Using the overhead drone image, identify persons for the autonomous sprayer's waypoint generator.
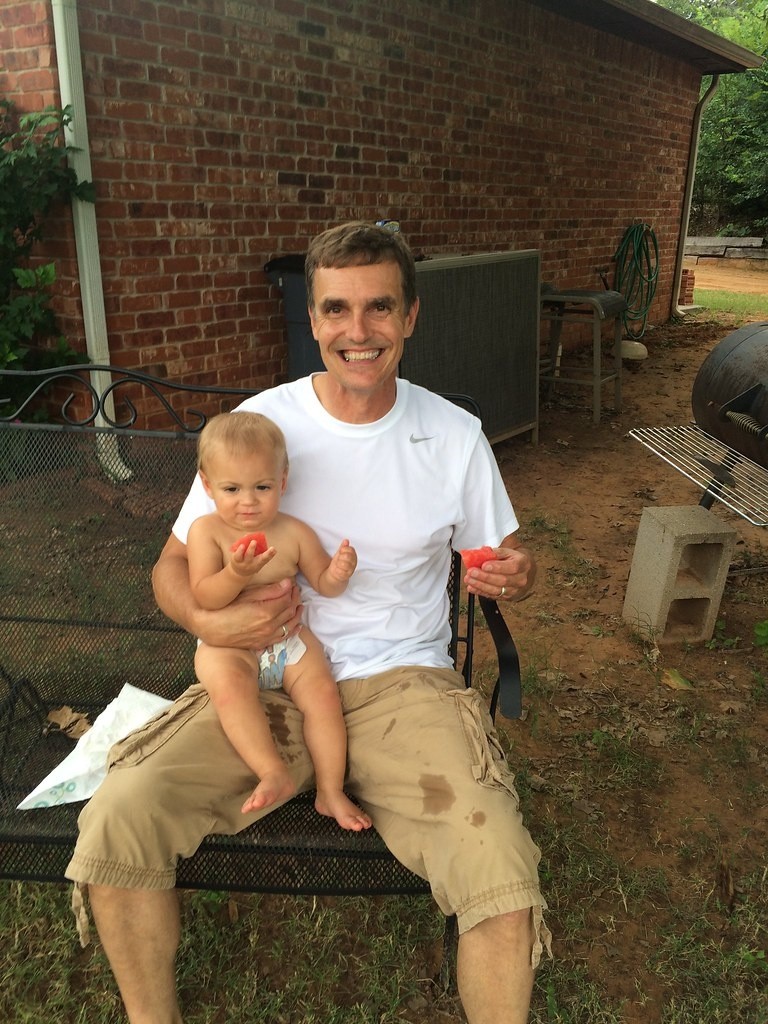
[63,222,556,1024]
[188,410,373,832]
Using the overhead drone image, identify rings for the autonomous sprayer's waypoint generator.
[498,587,506,596]
[281,625,290,638]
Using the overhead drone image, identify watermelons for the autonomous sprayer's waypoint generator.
[460,546,496,569]
[230,531,268,557]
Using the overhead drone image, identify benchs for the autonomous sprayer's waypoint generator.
[0,365,521,991]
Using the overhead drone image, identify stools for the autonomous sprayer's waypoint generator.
[541,287,627,421]
